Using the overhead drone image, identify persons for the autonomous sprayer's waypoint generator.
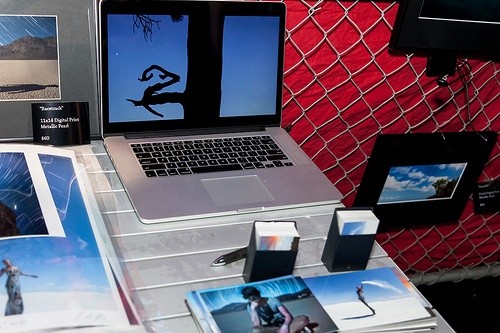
[356,284,376,314]
[0,258,38,317]
[241,286,310,333]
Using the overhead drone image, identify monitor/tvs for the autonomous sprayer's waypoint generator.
[387,0,500,62]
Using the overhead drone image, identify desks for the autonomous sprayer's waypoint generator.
[57,138,455,333]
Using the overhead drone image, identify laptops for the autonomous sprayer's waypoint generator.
[96,0,343,224]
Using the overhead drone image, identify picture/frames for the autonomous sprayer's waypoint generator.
[353,131,498,230]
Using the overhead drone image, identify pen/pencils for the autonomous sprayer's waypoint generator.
[210,244,249,268]
